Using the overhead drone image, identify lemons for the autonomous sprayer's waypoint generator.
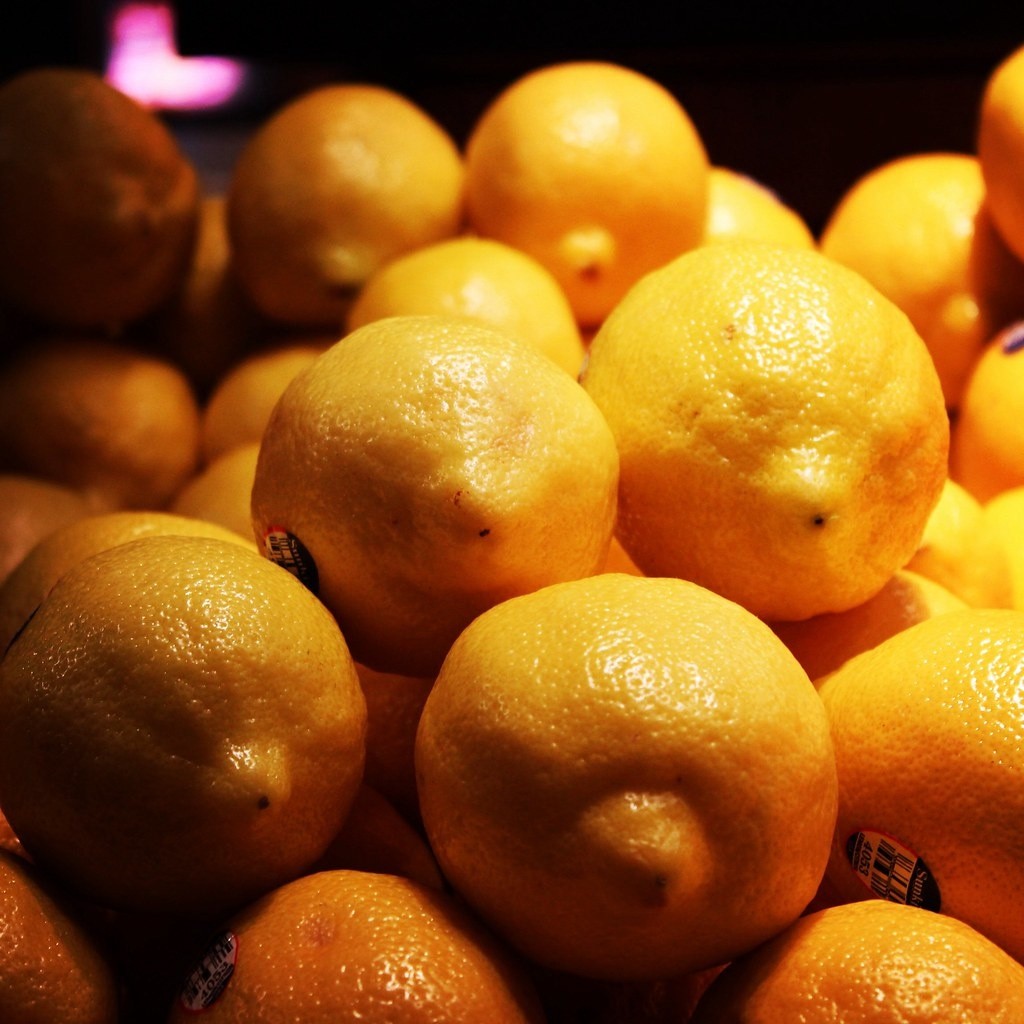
[0,44,1024,1024]
